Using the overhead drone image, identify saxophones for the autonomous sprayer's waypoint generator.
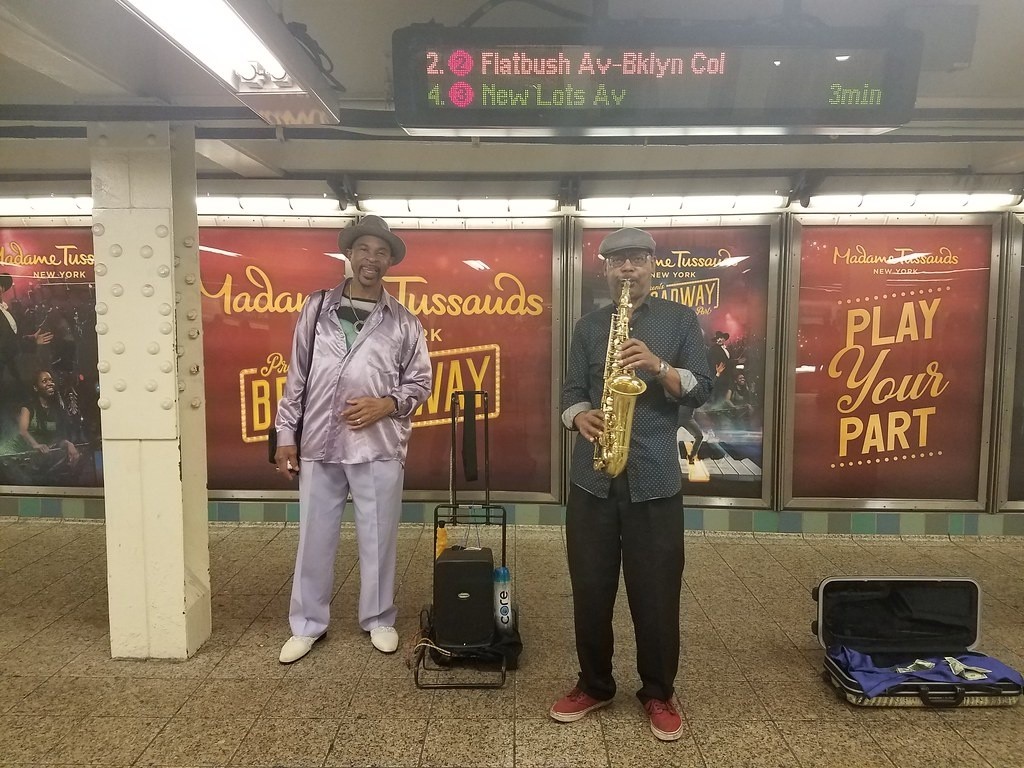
[593,280,648,477]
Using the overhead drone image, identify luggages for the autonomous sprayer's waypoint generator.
[811,575,1021,707]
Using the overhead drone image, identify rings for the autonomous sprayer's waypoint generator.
[357,419,362,425]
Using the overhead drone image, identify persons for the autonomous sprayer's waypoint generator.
[548,228,714,743]
[703,325,768,437]
[274,214,434,665]
[0,272,105,490]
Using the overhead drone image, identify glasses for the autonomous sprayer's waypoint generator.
[605,253,654,268]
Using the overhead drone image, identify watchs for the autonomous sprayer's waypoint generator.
[650,359,670,380]
[348,281,382,334]
[386,395,398,418]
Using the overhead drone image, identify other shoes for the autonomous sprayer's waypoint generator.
[370,625,399,652]
[279,631,327,663]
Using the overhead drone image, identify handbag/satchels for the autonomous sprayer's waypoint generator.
[268,407,304,466]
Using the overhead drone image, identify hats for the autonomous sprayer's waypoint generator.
[599,228,656,257]
[337,215,405,265]
[713,331,729,341]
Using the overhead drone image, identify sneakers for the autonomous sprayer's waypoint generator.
[550,685,613,721]
[642,696,683,740]
